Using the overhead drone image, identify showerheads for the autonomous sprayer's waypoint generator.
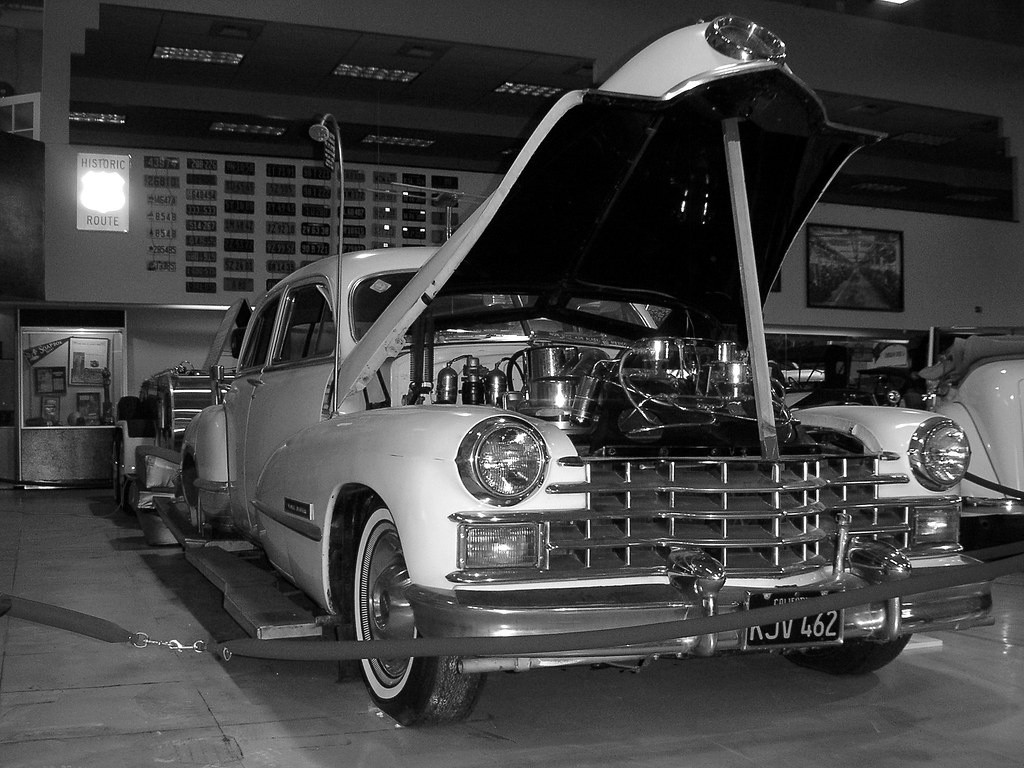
[308,110,351,420]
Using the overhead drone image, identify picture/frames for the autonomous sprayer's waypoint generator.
[68,337,110,386]
[805,221,906,313]
[41,395,61,423]
[34,366,67,396]
[78,392,101,417]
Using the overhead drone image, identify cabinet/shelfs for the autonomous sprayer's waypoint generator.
[15,306,129,488]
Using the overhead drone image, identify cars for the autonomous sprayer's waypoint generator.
[789,351,1024,528]
[178,26,994,731]
